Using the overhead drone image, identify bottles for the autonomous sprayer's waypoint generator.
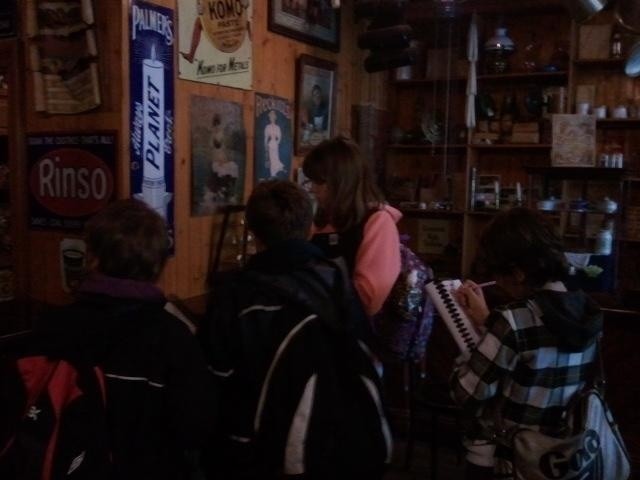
[611,33,624,56]
[595,228,613,255]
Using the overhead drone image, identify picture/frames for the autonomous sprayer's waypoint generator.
[268,0,341,53]
[294,54,338,157]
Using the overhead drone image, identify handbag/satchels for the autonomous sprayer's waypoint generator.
[497,386,635,480]
[348,207,436,364]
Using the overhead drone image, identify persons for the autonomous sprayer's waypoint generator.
[179,0,252,63]
[204,114,235,202]
[2,136,604,480]
[302,85,327,131]
[264,111,285,176]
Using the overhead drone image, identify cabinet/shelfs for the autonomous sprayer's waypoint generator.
[375,1,640,308]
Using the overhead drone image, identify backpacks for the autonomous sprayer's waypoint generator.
[254,257,395,480]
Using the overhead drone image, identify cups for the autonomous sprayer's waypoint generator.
[577,101,589,114]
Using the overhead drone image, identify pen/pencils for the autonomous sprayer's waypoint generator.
[453,281,497,296]
